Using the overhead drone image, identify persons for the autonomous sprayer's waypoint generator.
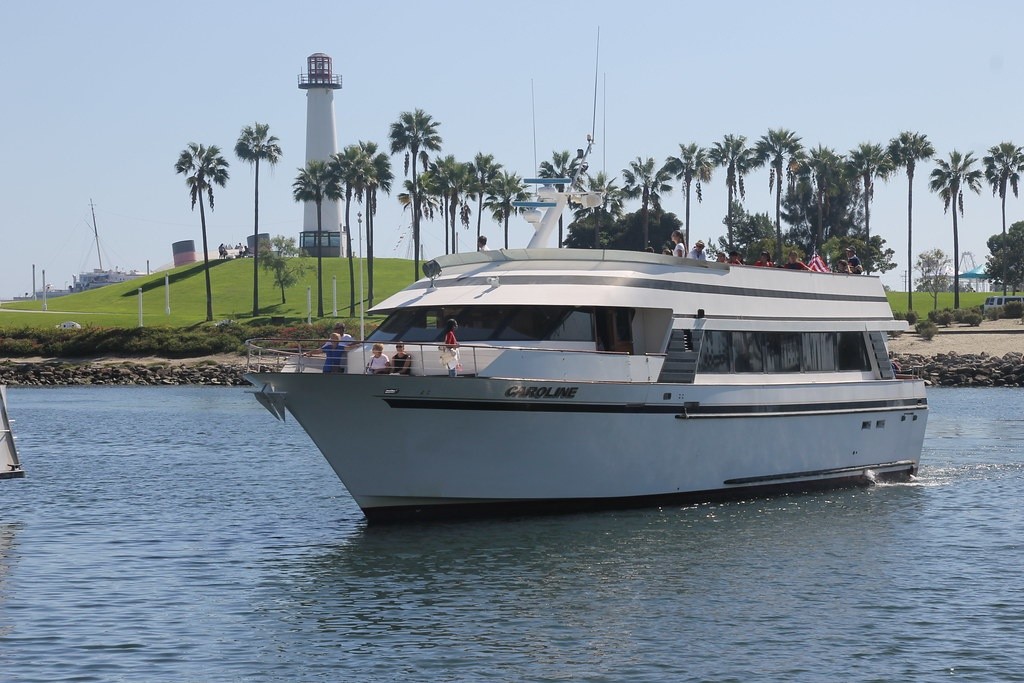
[368,343,390,375]
[444,319,460,377]
[321,323,354,374]
[477,229,863,277]
[391,341,413,375]
[306,332,363,374]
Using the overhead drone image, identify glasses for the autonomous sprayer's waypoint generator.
[696,245,701,247]
[396,345,402,348]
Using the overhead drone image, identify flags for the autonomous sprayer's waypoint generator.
[807,252,831,273]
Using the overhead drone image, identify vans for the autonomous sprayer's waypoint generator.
[983,296,1024,319]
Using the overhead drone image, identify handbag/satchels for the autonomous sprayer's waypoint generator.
[438,345,453,365]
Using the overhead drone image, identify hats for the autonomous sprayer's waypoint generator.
[695,240,705,248]
[845,245,856,254]
[761,251,770,262]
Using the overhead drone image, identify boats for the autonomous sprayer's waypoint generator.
[239,130,932,526]
